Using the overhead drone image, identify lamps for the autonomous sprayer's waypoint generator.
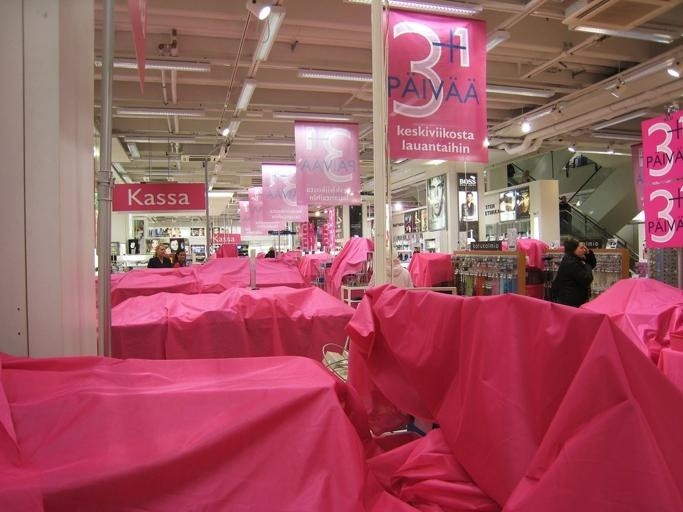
[204,1,290,193]
[362,53,681,192]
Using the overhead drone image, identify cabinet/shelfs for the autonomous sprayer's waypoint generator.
[450,251,526,296]
[540,248,631,301]
[481,180,560,245]
[391,206,428,264]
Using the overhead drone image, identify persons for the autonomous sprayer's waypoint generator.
[421,209,428,232]
[172,248,186,268]
[559,195,573,233]
[146,244,171,268]
[428,175,446,230]
[461,192,475,217]
[412,211,421,232]
[549,237,597,308]
[521,169,530,184]
